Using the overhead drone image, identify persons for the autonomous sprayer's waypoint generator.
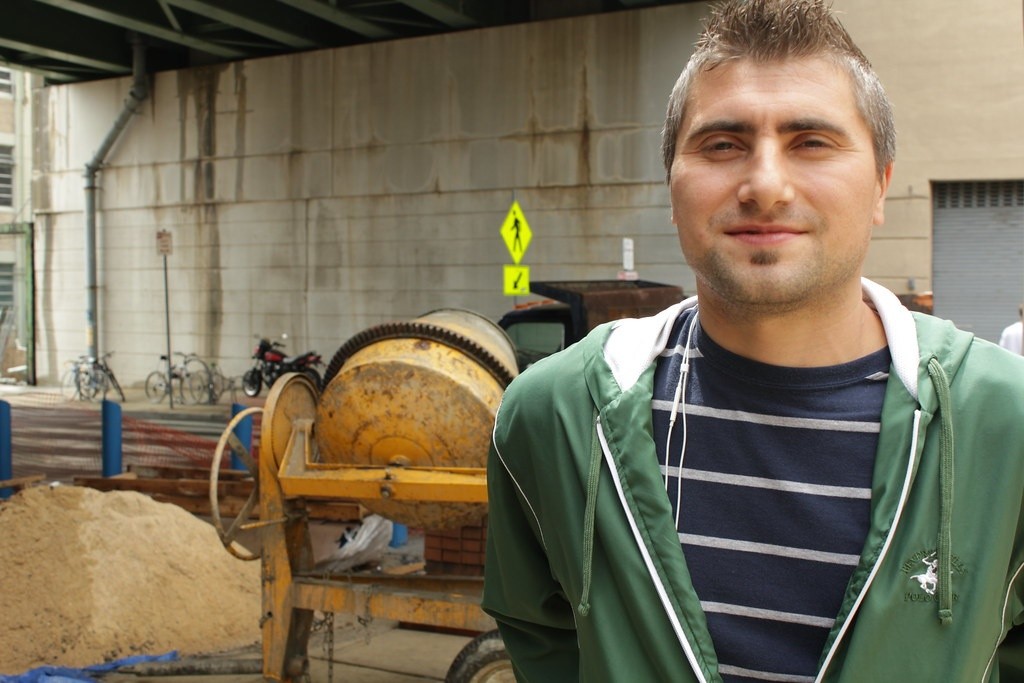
[999,305,1024,357]
[480,1,1024,683]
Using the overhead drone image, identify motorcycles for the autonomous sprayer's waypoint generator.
[241,332,324,389]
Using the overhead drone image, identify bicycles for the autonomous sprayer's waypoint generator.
[60,351,125,402]
[143,351,245,405]
